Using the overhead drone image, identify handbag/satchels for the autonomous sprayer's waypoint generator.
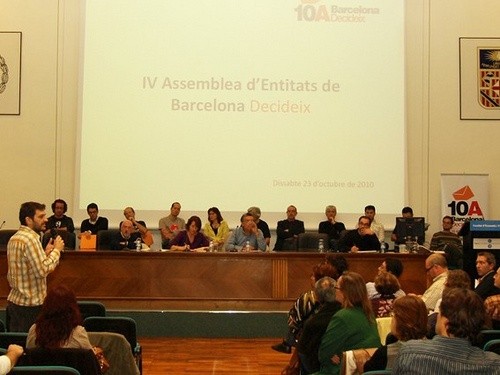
[93,345,110,372]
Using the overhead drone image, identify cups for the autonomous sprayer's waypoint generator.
[399,244,406,253]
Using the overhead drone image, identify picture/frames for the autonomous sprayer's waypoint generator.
[459,36,500,120]
[0,30,22,116]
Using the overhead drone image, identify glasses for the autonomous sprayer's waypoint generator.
[425,263,435,272]
[334,286,341,291]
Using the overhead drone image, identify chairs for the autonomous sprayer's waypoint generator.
[0,229,20,246]
[25,346,110,375]
[7,365,81,375]
[76,300,105,320]
[43,229,75,249]
[82,316,143,375]
[291,232,329,249]
[475,330,500,353]
[97,229,141,249]
[0,331,28,349]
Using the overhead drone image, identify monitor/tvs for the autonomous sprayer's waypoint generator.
[396,217,425,245]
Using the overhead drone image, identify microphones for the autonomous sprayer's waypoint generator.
[0,221,5,229]
[50,228,64,253]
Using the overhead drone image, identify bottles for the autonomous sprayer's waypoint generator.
[136,241,141,252]
[245,241,250,254]
[318,239,324,254]
[380,241,386,254]
[209,241,214,253]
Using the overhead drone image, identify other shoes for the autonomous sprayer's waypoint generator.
[271,343,291,354]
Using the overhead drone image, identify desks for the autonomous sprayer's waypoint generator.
[0,248,434,311]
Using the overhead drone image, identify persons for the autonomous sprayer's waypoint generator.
[365,205,385,244]
[26,287,110,375]
[224,212,267,253]
[203,207,230,248]
[240,206,271,247]
[271,244,500,354]
[158,202,187,249]
[430,216,463,251]
[363,295,432,373]
[389,287,500,375]
[44,199,153,249]
[311,270,381,375]
[391,206,429,242]
[318,204,345,240]
[272,205,305,251]
[0,344,24,375]
[167,215,209,251]
[336,216,381,252]
[281,276,343,375]
[6,202,65,333]
